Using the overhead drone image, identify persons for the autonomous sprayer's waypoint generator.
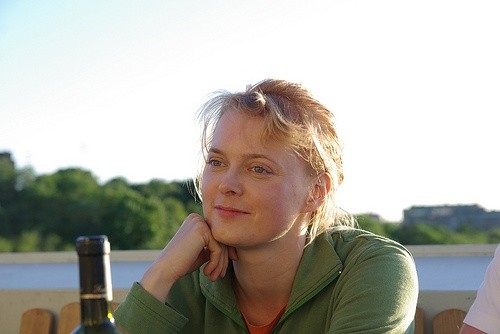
[459,242,500,334]
[112,79,420,334]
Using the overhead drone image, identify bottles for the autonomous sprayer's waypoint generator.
[71,235,121,334]
